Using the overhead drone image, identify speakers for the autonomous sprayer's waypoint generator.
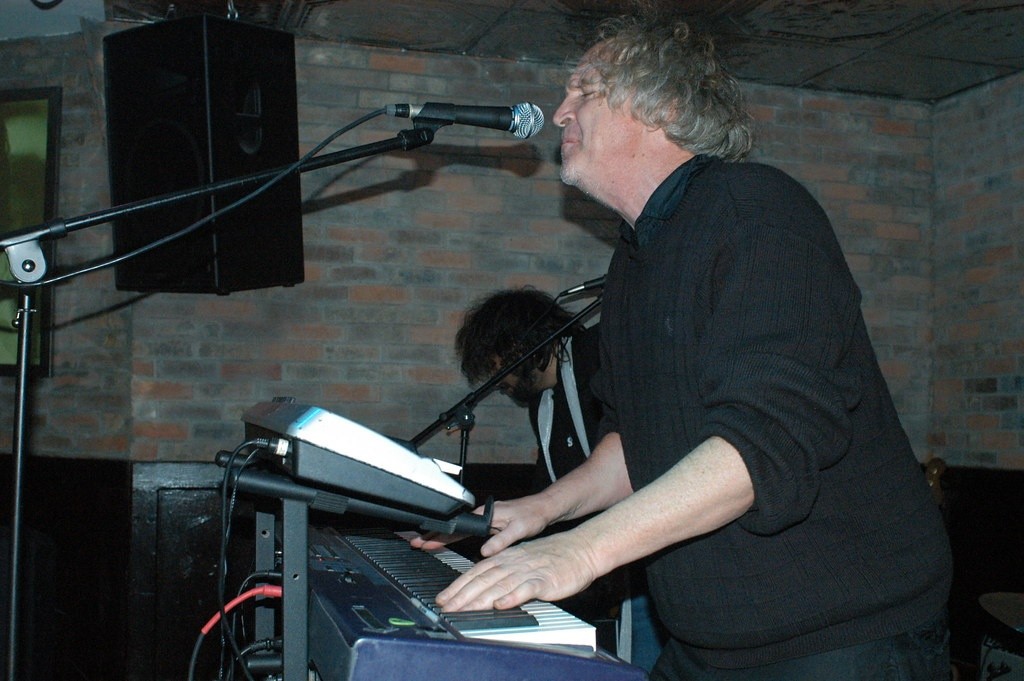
[103,14,305,297]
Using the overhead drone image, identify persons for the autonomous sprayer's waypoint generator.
[455,287,673,678]
[408,20,957,681]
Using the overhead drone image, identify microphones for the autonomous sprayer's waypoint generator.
[385,100,545,140]
[557,275,610,299]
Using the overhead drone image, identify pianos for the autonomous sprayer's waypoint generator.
[270,525,653,681]
